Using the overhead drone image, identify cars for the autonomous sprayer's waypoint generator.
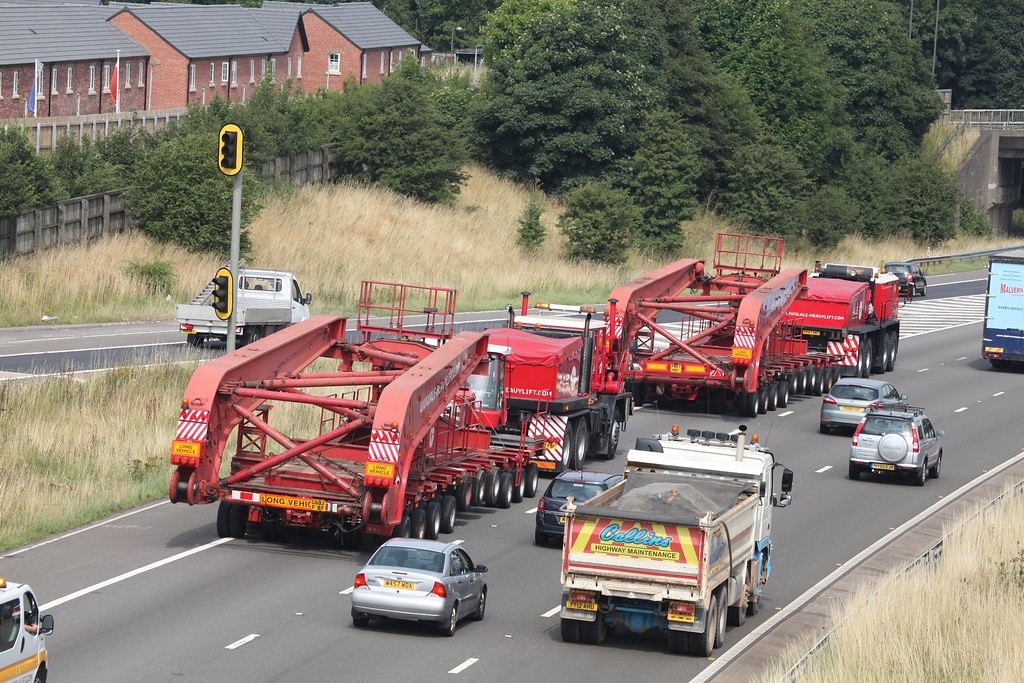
[820,378,908,434]
[884,262,927,296]
[351,536,489,636]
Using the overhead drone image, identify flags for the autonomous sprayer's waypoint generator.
[109,61,118,105]
[27,78,35,114]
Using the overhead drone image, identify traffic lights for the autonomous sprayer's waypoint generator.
[211,268,234,321]
[218,123,243,176]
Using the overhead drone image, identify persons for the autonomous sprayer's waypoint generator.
[24,623,38,633]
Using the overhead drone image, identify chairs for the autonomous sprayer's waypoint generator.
[381,557,398,566]
[426,563,441,573]
[254,285,262,290]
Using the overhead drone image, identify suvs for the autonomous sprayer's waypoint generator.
[849,403,944,488]
[535,470,624,545]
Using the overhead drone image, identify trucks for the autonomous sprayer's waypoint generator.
[981,249,1024,370]
[175,268,312,349]
[0,578,55,683]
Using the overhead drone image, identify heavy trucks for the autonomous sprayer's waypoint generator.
[560,424,794,658]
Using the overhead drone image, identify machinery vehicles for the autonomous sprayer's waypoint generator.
[611,231,901,417]
[168,279,632,549]
